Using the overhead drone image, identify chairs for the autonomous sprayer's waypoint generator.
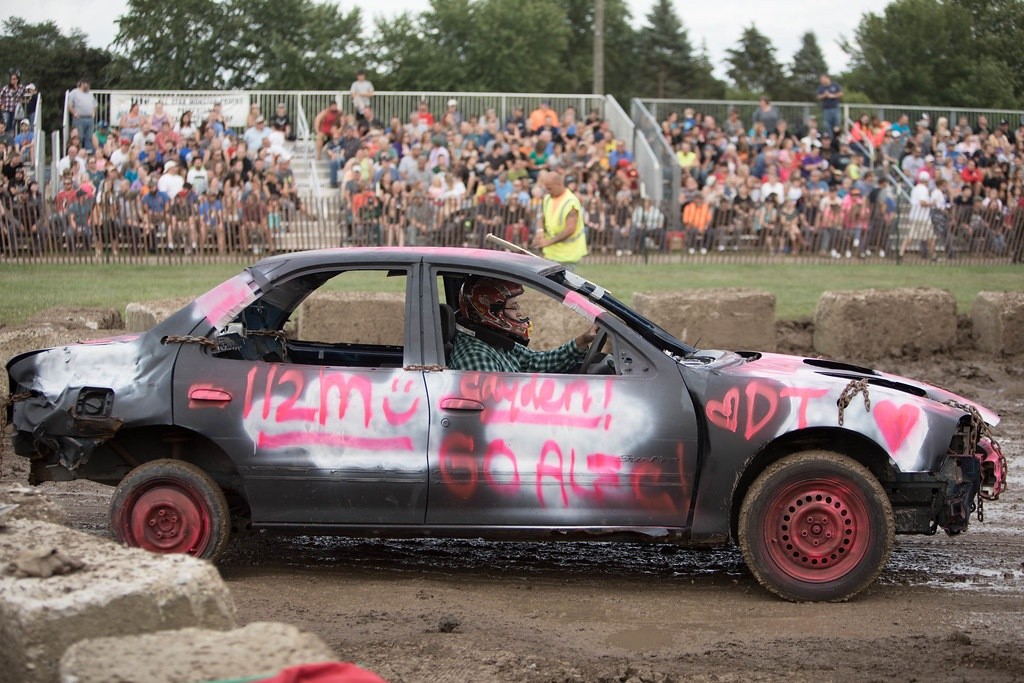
[440,303,456,361]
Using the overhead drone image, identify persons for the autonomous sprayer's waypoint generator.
[815,75,847,134]
[0,70,536,263]
[538,101,632,169]
[641,93,1023,266]
[530,170,590,276]
[566,170,668,255]
[449,271,604,375]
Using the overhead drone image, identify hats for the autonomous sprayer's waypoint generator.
[163,161,177,173]
[919,171,930,182]
[447,100,458,106]
[21,118,30,125]
[121,139,131,145]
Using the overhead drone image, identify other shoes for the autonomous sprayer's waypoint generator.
[865,249,872,256]
[853,238,859,247]
[616,249,622,256]
[837,253,841,258]
[718,244,724,251]
[701,247,707,255]
[845,249,851,258]
[626,250,632,256]
[879,250,885,258]
[688,247,695,254]
[831,249,837,258]
[859,252,865,258]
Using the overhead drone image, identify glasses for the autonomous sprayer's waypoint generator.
[145,141,153,145]
[64,183,70,185]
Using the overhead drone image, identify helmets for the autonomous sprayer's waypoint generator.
[459,275,532,347]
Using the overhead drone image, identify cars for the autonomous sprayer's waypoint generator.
[3,233,1008,603]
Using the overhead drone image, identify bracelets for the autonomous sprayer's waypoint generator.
[536,228,545,233]
